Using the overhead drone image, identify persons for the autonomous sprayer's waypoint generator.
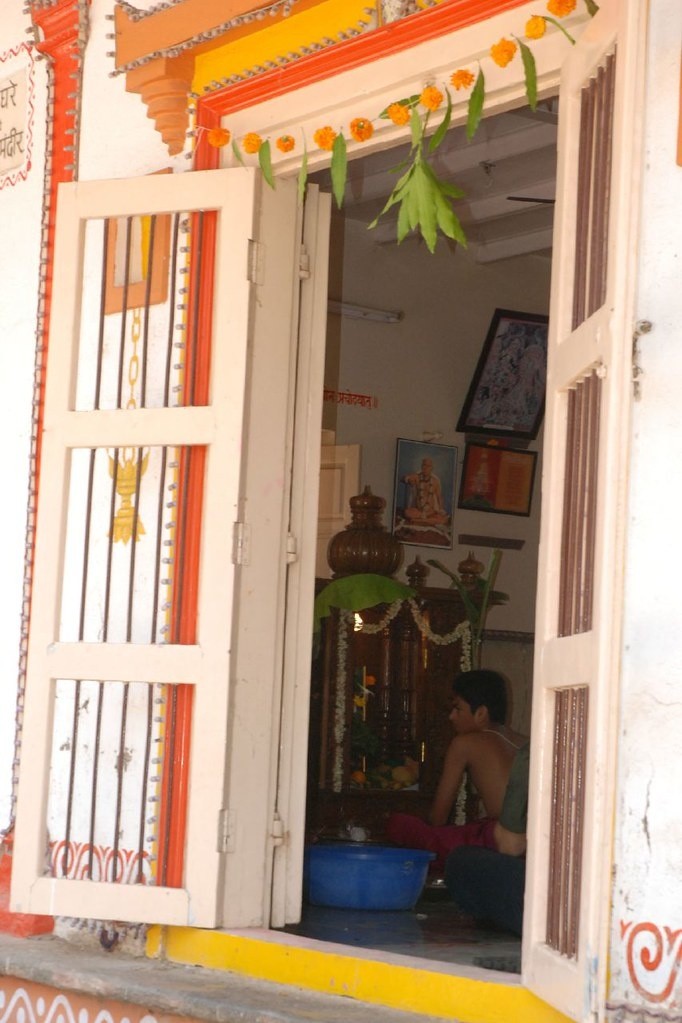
[384,668,530,883]
[447,735,530,937]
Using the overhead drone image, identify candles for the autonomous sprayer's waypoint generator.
[421,740,425,763]
[363,756,366,773]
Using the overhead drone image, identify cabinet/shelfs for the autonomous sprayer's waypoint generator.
[305,576,510,847]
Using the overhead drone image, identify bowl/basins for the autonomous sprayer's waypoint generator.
[304,844,436,911]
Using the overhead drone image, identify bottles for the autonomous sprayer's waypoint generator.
[327,484,403,579]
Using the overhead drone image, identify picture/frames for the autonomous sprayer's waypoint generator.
[392,437,459,550]
[455,309,548,441]
[456,442,538,517]
